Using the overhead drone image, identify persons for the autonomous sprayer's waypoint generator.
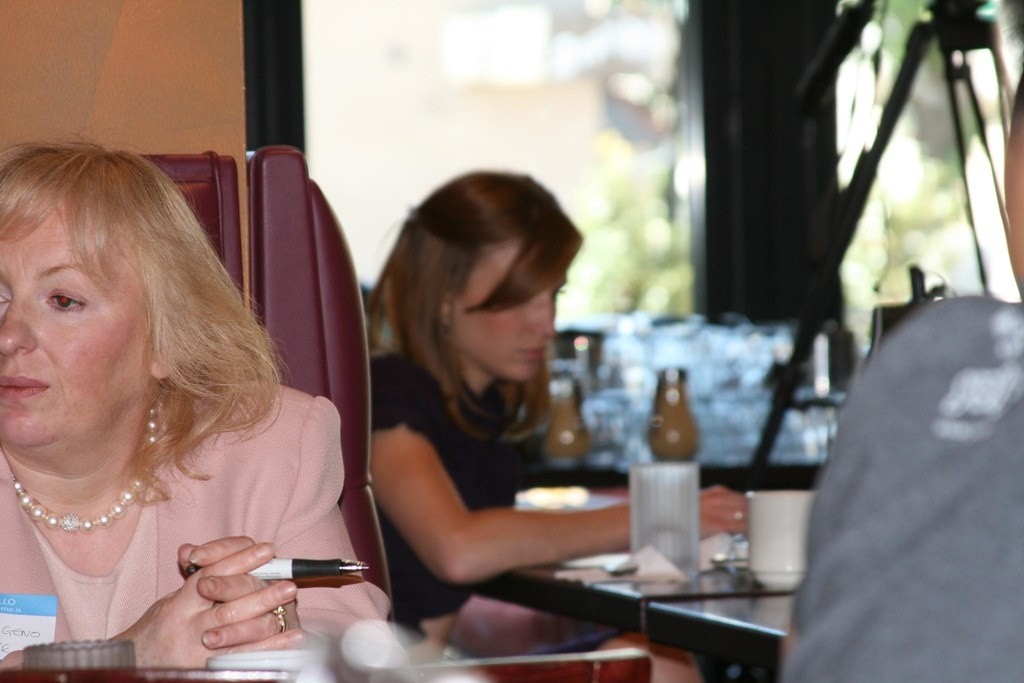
[1,146,393,673]
[773,49,1023,682]
[368,170,752,683]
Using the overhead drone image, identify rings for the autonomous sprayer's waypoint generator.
[733,509,742,521]
[268,605,288,633]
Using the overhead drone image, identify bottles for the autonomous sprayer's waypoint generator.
[541,372,588,459]
[644,365,699,461]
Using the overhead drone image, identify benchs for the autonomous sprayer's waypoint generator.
[245,141,655,683]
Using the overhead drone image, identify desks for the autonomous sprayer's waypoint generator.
[477,485,824,683]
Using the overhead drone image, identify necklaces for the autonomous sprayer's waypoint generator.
[10,407,159,534]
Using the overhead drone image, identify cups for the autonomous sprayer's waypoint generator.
[745,490,816,593]
[629,460,699,582]
[22,639,136,670]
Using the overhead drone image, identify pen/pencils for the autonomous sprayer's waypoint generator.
[186,558,370,579]
